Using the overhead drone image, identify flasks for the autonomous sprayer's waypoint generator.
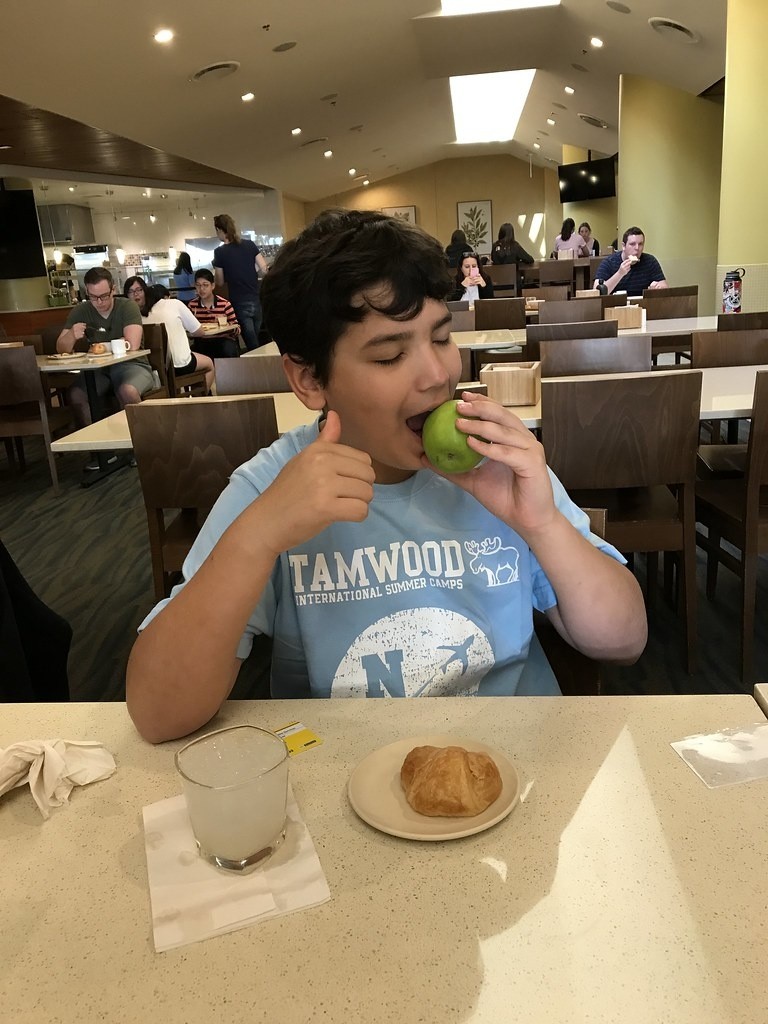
[722,268,746,314]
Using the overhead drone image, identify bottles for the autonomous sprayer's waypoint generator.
[595,279,608,296]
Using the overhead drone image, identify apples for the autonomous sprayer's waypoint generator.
[422,399,494,475]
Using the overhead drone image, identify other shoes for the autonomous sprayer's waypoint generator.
[85,454,117,470]
[131,461,137,466]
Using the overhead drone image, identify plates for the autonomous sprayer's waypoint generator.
[87,352,111,357]
[47,353,86,359]
[345,734,520,842]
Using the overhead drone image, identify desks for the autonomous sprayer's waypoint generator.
[0,695,768,1024]
[239,328,515,357]
[508,315,717,346]
[35,348,152,486]
[188,323,238,337]
[51,364,768,454]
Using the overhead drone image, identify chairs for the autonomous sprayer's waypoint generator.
[0,251,768,695]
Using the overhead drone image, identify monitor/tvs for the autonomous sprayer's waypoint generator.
[558,157,616,203]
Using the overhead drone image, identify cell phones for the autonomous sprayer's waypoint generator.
[470,268,479,279]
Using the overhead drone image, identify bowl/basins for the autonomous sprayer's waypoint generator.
[528,300,545,310]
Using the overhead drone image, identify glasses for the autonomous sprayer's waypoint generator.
[194,283,209,290]
[127,287,143,297]
[86,287,112,301]
[462,251,478,258]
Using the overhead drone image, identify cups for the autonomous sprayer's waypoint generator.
[111,339,130,355]
[175,724,291,875]
[218,317,227,326]
[526,297,536,305]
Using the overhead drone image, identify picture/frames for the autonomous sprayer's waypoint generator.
[457,200,494,255]
[380,206,416,224]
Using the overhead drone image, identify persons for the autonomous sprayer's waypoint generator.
[447,252,494,309]
[148,284,214,398]
[554,217,589,259]
[125,205,648,747]
[578,223,599,257]
[491,223,534,297]
[124,276,150,317]
[446,230,474,268]
[174,252,196,306]
[212,214,266,350]
[47,253,75,302]
[592,227,668,297]
[188,269,241,365]
[56,267,154,472]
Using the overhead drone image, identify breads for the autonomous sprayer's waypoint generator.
[628,255,639,261]
[92,344,106,354]
[400,745,503,818]
